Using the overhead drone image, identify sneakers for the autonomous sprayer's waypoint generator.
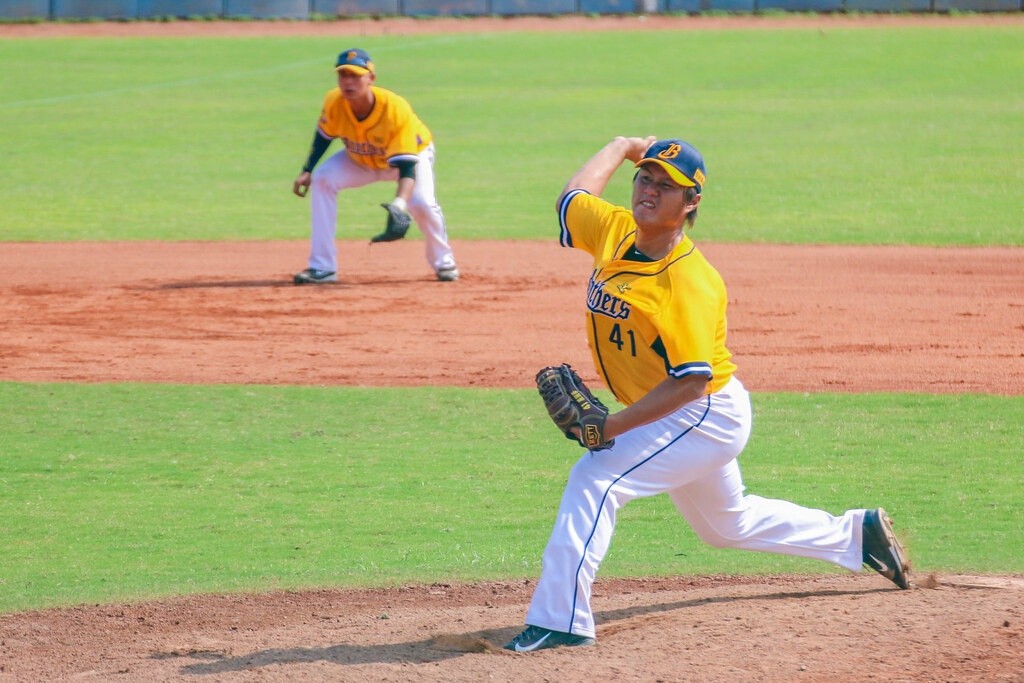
[862,507,911,589]
[503,626,596,652]
[294,268,337,283]
[437,266,458,281]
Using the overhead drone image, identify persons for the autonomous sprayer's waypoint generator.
[502,136,912,653]
[293,48,458,282]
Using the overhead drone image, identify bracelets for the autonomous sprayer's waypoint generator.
[392,198,406,210]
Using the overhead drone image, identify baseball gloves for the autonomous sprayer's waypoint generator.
[533,360,618,459]
[368,202,412,248]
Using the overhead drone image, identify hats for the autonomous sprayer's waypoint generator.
[334,49,374,75]
[635,138,706,194]
